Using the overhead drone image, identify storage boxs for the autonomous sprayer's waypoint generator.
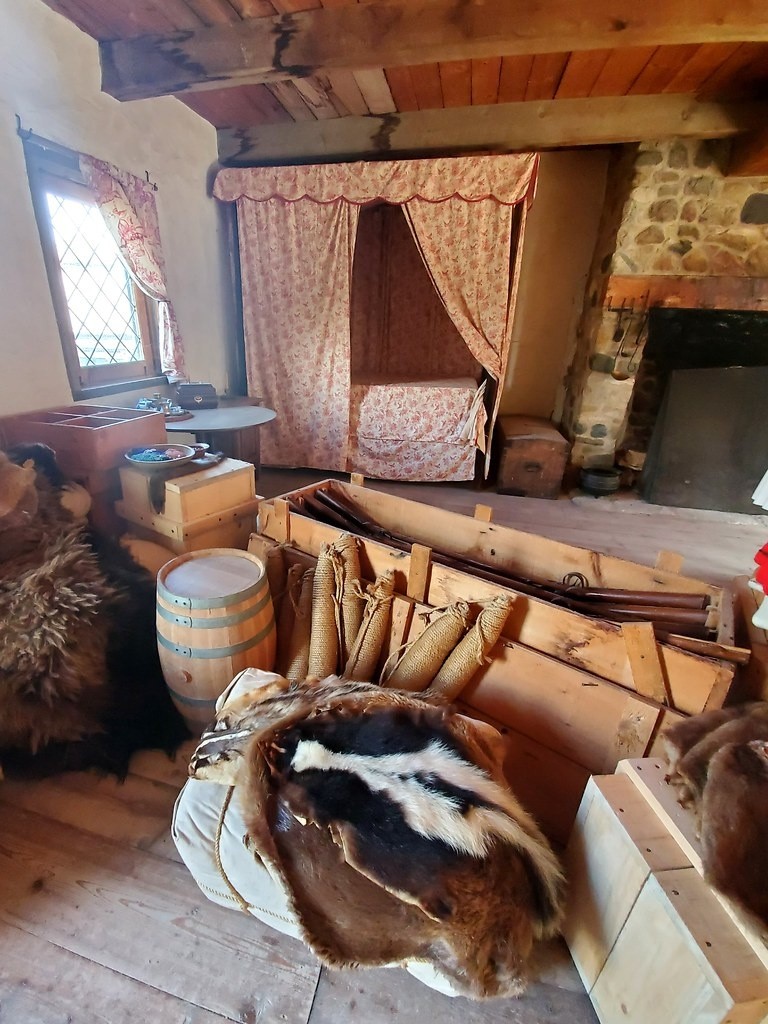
[244,476,735,835]
[0,404,264,581]
[561,757,768,1023]
[175,382,218,410]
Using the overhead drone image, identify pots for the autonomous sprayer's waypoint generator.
[619,459,642,489]
[580,465,622,499]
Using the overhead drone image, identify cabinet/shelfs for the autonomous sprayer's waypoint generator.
[206,395,264,479]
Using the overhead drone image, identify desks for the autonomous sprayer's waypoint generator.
[165,402,276,453]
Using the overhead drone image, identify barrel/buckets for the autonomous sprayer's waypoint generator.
[157,548,277,733]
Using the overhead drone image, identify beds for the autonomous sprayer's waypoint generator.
[212,149,541,482]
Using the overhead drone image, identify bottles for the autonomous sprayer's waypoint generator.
[137,393,184,417]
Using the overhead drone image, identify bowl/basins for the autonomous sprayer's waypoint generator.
[184,443,209,458]
[125,444,195,470]
